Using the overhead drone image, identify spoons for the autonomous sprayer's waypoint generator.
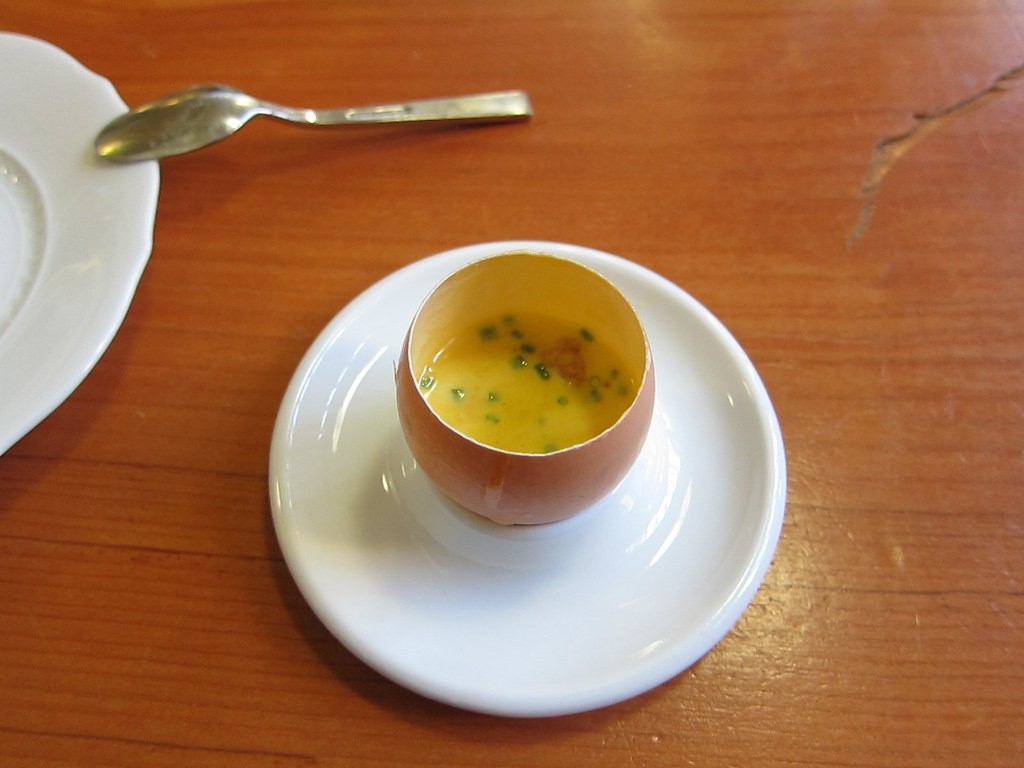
[93,82,534,162]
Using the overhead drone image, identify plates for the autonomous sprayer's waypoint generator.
[0,32,160,458]
[268,240,789,719]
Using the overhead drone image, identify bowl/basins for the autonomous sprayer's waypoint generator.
[396,249,655,527]
